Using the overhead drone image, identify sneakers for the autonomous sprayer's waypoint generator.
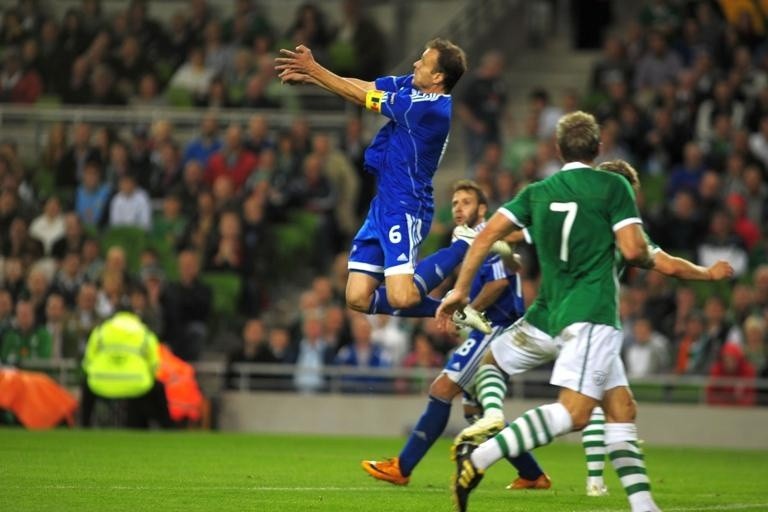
[450,413,505,512]
[441,288,493,335]
[451,223,512,257]
[506,473,552,491]
[586,485,608,496]
[361,457,411,486]
